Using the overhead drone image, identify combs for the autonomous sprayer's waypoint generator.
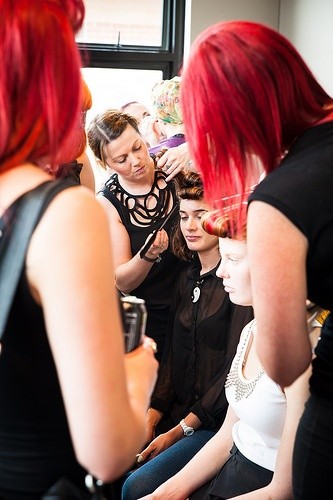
[140,204,178,259]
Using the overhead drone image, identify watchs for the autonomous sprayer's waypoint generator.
[180,418,194,437]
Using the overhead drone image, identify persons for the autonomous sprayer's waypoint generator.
[151,76,184,125]
[139,199,323,500]
[179,21,333,500]
[118,101,166,150]
[122,149,254,500]
[0,0,159,500]
[87,108,195,364]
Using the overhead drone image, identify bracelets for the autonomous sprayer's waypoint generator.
[139,250,158,263]
